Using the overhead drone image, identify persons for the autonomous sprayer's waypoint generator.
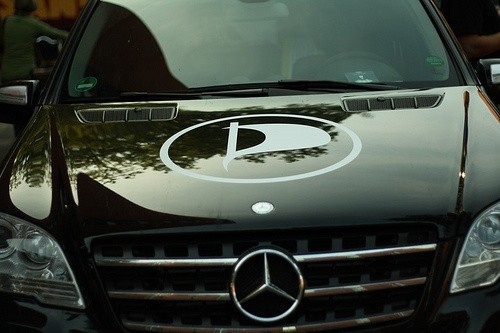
[0,0,72,137]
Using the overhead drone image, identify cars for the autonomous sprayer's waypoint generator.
[0,1,500,333]
